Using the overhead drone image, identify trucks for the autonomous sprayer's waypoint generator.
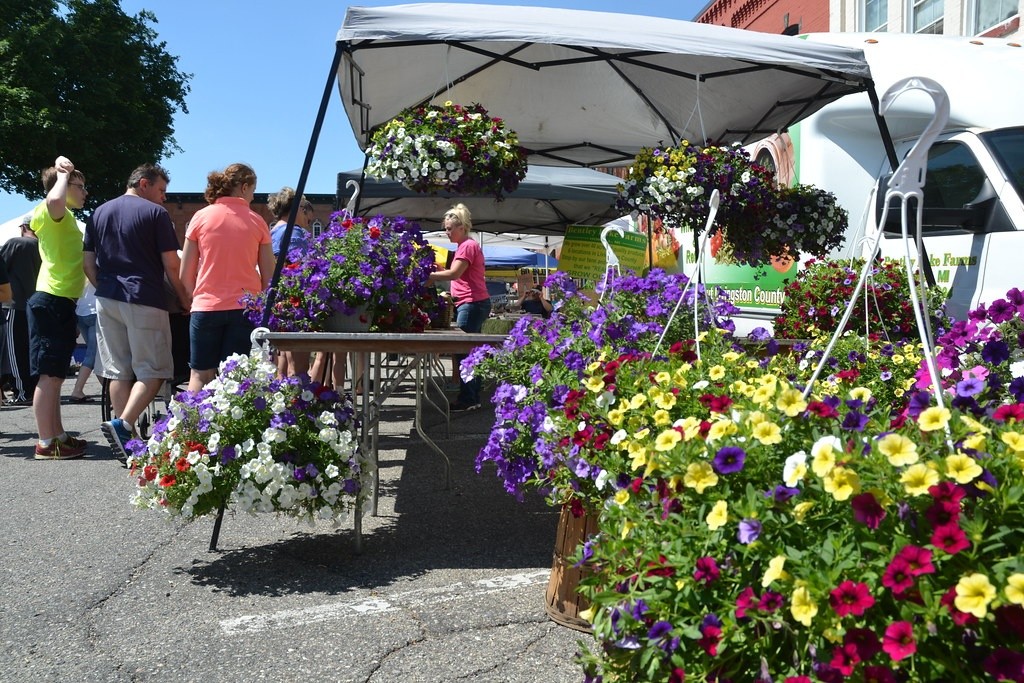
[659,30,1024,382]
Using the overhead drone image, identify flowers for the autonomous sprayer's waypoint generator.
[125,100,1024,683]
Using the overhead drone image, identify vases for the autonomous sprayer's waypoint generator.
[426,304,452,329]
[545,500,634,633]
[323,292,376,332]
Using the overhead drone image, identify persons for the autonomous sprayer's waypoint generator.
[427,203,492,412]
[178,163,275,394]
[261,186,313,379]
[0,215,41,409]
[84,164,191,467]
[70,273,99,406]
[311,351,364,396]
[514,284,554,320]
[26,156,88,461]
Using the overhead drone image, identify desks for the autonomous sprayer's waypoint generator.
[254,326,531,553]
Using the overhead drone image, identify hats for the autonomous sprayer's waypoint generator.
[18,215,33,227]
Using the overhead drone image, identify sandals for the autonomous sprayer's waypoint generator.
[69,395,95,403]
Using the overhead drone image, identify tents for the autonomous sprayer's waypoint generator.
[423,240,538,284]
[0,199,87,246]
[336,165,655,272]
[209,3,955,550]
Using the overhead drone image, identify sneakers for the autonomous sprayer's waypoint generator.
[450,400,481,413]
[100,418,133,464]
[35,432,88,460]
[9,399,33,406]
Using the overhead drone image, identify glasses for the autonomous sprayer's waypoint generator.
[68,183,85,190]
[300,205,307,212]
